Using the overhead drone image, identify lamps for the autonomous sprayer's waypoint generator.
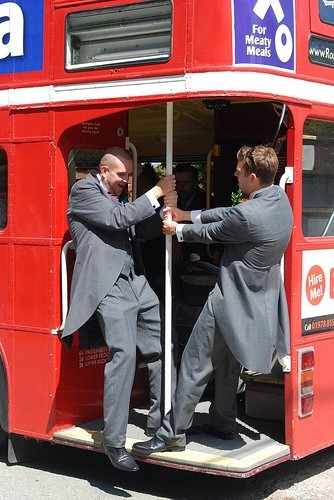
[202,99,230,111]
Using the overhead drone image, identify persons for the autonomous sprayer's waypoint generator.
[121,163,214,331]
[132,146,294,452]
[62,146,178,472]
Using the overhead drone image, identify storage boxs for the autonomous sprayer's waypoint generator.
[245,382,285,423]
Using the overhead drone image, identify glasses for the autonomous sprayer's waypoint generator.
[245,146,260,178]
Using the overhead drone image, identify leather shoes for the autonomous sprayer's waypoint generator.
[101,443,139,471]
[145,424,199,434]
[202,423,233,440]
[132,436,185,453]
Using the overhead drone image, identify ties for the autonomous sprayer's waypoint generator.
[108,192,123,207]
